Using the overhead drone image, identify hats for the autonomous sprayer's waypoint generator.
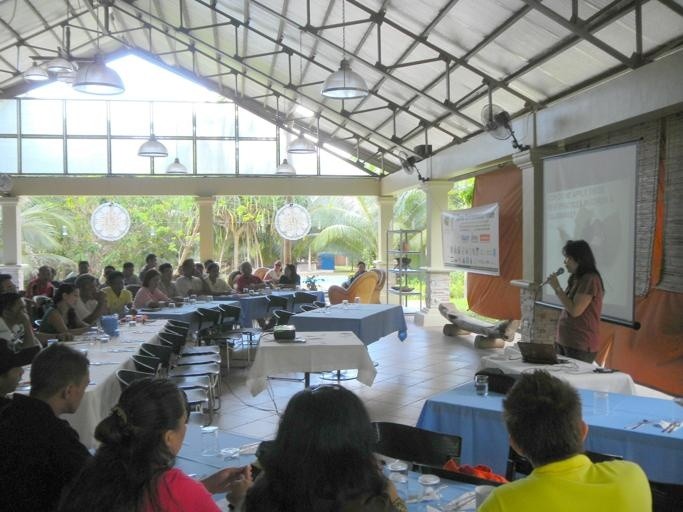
[0,338,40,374]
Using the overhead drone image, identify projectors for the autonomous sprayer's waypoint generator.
[273,324,296,341]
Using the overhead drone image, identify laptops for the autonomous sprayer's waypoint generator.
[517,341,569,365]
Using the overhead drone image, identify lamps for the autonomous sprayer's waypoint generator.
[275,87,295,174]
[167,135,189,175]
[41,23,77,74]
[138,114,168,157]
[287,27,315,154]
[72,1,126,97]
[322,1,372,100]
[24,48,47,82]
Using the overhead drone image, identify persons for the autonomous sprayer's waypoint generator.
[0,293,43,351]
[57,377,253,511]
[122,262,137,285]
[62,261,99,289]
[205,263,234,296]
[0,343,94,512]
[475,369,653,512]
[75,274,107,326]
[26,265,56,298]
[233,261,265,292]
[548,240,605,364]
[280,263,300,288]
[100,271,133,314]
[0,338,40,405]
[203,260,213,276]
[239,382,407,511]
[175,258,203,297]
[193,262,203,277]
[265,260,283,283]
[0,274,71,342]
[100,266,115,288]
[39,284,97,334]
[139,253,157,281]
[173,266,183,281]
[134,267,183,308]
[341,262,368,289]
[158,263,177,299]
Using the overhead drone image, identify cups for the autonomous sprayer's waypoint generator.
[207,295,213,305]
[594,391,609,414]
[191,295,196,300]
[200,426,221,457]
[387,459,409,503]
[126,314,133,326]
[343,299,348,311]
[46,338,58,345]
[184,297,189,305]
[266,287,271,294]
[159,301,164,311]
[169,303,176,314]
[136,315,145,331]
[87,332,97,347]
[129,320,136,334]
[102,338,110,354]
[244,288,249,294]
[250,290,254,297]
[419,475,441,512]
[475,374,490,397]
[190,299,197,309]
[355,297,361,310]
[115,329,122,343]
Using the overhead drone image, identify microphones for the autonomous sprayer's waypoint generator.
[537,266,565,290]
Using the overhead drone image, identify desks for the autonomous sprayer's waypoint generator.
[426,375,682,488]
[481,341,631,394]
[85,420,488,512]
[257,330,364,387]
[139,299,239,338]
[289,301,402,381]
[6,319,167,454]
[213,288,324,324]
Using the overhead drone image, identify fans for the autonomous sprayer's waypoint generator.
[398,150,430,181]
[481,103,530,152]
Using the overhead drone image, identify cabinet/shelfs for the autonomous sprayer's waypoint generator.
[387,230,422,315]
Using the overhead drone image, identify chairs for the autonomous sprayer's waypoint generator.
[158,332,221,375]
[297,304,317,313]
[194,307,220,346]
[313,300,325,308]
[162,324,221,373]
[218,303,240,323]
[114,370,213,426]
[271,309,295,325]
[328,273,378,304]
[369,268,385,292]
[265,295,287,312]
[131,355,215,413]
[167,320,221,354]
[291,291,316,313]
[139,343,220,390]
